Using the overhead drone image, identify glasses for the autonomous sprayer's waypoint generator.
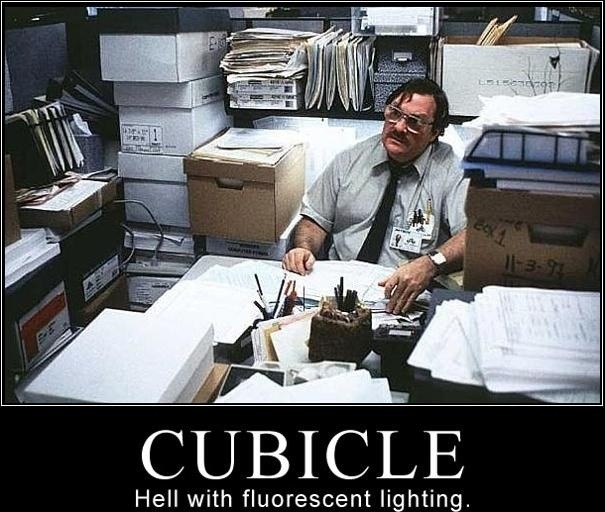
[383,102,435,138]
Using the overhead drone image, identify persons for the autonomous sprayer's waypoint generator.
[281,79,470,315]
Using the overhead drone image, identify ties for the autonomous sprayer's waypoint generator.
[352,162,416,265]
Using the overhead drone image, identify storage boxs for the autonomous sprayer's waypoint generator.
[461,179,599,297]
[2,4,306,403]
[441,38,598,118]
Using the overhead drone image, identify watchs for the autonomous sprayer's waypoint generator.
[427,249,449,276]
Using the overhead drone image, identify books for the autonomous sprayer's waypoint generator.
[220,27,378,115]
[476,15,518,46]
[250,305,323,367]
[286,256,434,315]
[1,65,120,208]
[455,91,601,201]
[404,284,603,406]
[191,126,306,168]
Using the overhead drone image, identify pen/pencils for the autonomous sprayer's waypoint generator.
[334,277,359,312]
[250,273,306,320]
